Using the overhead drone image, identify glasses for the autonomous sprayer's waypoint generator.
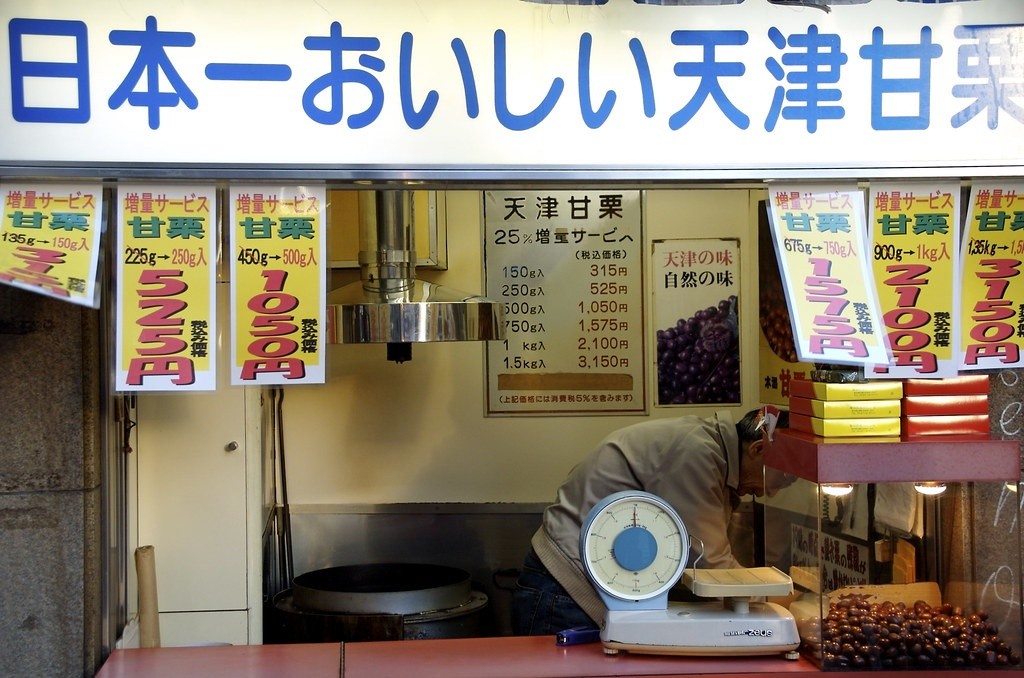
[778,470,793,489]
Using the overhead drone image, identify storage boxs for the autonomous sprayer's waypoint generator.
[787,374,989,439]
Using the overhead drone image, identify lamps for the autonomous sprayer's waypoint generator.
[821,482,853,497]
[914,482,947,495]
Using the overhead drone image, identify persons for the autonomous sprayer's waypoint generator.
[512,409,799,636]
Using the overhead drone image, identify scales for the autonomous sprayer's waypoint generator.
[578,489,803,661]
